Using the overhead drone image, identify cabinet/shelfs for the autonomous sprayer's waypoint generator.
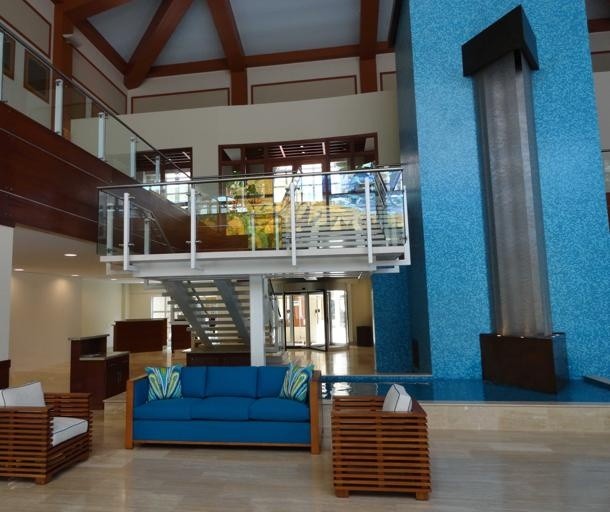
[69,333,130,410]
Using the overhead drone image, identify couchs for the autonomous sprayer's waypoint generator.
[124,366,323,455]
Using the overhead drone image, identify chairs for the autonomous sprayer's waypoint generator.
[1,380,95,486]
[242,166,303,250]
[329,384,432,501]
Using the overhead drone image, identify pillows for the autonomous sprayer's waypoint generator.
[144,362,184,402]
[279,362,315,401]
